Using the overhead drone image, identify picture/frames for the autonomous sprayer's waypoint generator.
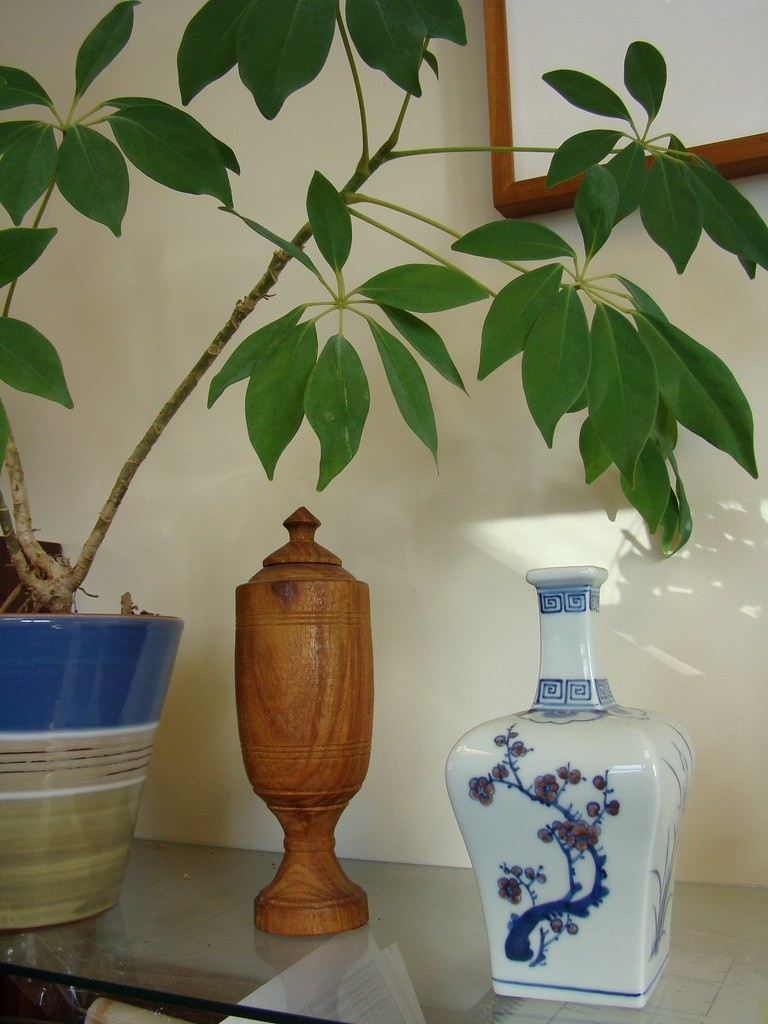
[482,0,768,217]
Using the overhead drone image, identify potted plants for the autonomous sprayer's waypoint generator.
[0,0,768,931]
